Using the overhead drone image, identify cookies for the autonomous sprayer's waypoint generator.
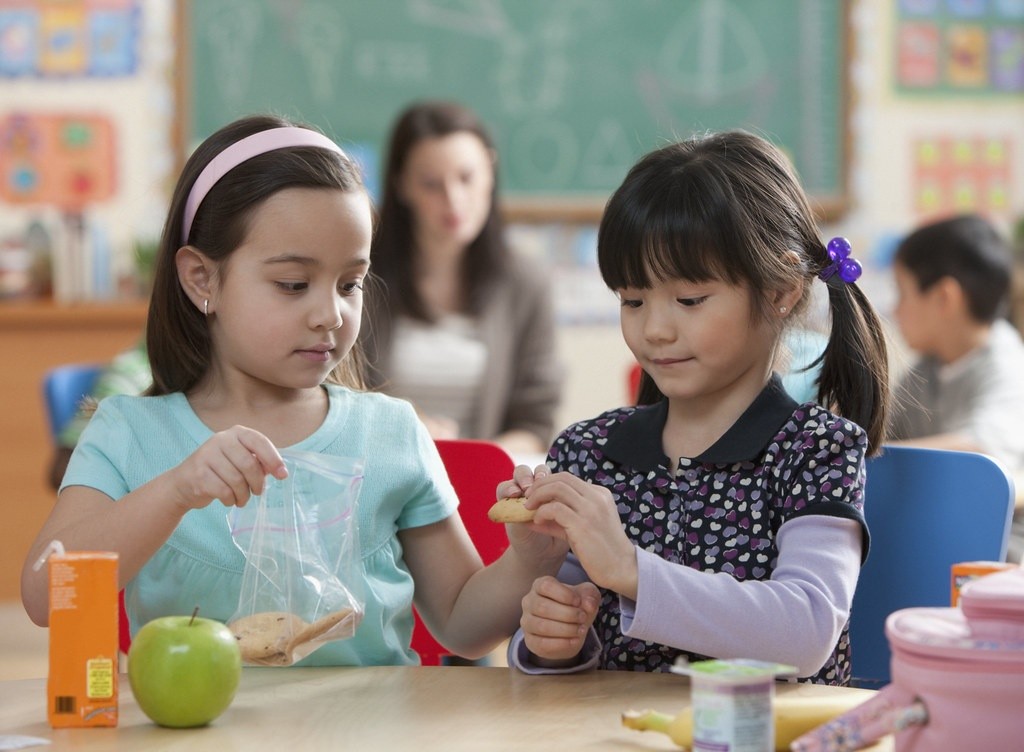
[225,612,301,657]
[286,609,351,653]
[243,621,308,666]
[487,497,540,523]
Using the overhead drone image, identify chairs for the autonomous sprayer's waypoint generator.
[852,448,1015,692]
[118,439,520,667]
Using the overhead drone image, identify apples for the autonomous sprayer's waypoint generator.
[129,607,240,727]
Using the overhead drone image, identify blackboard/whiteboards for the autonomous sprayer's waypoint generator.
[176,3,853,220]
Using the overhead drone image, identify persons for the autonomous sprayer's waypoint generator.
[355,96,567,458]
[18,113,571,670]
[504,126,892,687]
[882,208,1024,563]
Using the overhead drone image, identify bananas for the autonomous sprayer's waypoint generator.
[620,703,883,751]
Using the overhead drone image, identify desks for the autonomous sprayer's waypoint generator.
[0,668,695,752]
[0,292,189,600]
